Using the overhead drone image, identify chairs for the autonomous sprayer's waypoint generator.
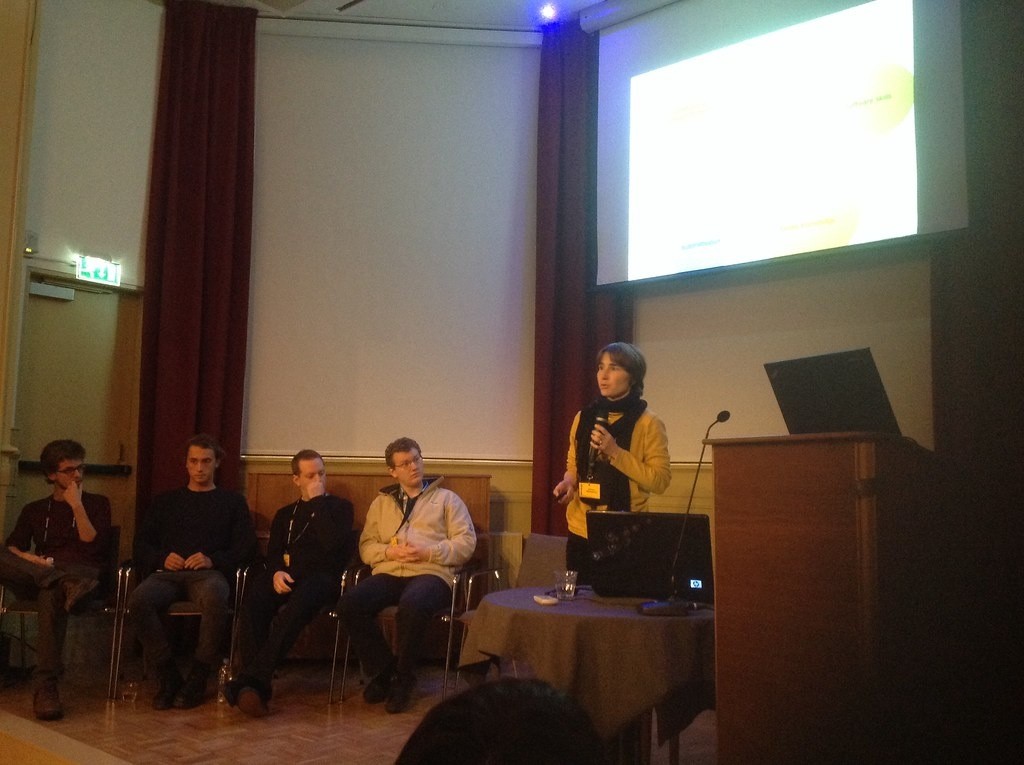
[339,523,476,705]
[229,528,362,705]
[113,509,258,697]
[0,524,130,699]
[455,532,570,697]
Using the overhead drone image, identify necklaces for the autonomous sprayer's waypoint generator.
[283,493,328,567]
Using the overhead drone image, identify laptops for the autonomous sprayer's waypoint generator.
[763,346,902,437]
[585,510,714,605]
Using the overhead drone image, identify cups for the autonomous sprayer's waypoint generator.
[122,681,139,703]
[552,571,577,600]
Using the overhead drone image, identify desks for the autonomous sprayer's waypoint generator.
[459,582,717,765]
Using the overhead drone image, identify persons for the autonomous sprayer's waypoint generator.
[0,438,113,721]
[552,341,672,586]
[342,437,478,713]
[218,450,355,717]
[124,435,257,710]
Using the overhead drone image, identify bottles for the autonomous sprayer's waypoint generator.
[218,659,233,703]
[46,557,53,565]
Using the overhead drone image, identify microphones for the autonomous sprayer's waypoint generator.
[590,408,609,463]
[638,410,732,616]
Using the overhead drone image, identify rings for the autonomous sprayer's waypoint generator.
[600,440,603,444]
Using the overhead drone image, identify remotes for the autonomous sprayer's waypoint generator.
[534,595,558,606]
[553,491,567,503]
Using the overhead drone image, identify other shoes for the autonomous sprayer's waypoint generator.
[224,680,268,718]
[152,671,207,709]
[383,670,417,715]
[32,683,66,720]
[363,671,391,704]
[64,576,100,614]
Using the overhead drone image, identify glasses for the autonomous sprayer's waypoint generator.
[394,454,423,468]
[57,463,88,474]
